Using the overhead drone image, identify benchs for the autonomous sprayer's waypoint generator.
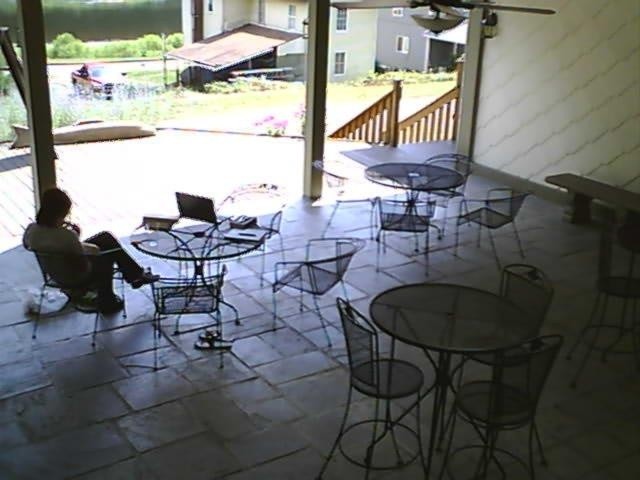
[544,174,640,225]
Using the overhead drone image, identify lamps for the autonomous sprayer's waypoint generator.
[481,11,499,39]
[410,7,464,35]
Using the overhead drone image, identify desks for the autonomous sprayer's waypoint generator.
[364,163,466,245]
[131,222,265,332]
[363,283,538,475]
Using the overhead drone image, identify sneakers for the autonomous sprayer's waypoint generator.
[132,272,160,288]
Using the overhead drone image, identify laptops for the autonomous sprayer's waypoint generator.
[176,192,234,225]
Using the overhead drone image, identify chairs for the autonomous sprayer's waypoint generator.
[30,249,103,350]
[311,295,425,480]
[419,150,473,235]
[566,218,640,391]
[497,264,554,338]
[275,239,364,347]
[147,267,226,367]
[444,332,564,478]
[459,186,534,267]
[312,158,382,240]
[209,184,288,288]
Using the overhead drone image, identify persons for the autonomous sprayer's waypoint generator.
[22,188,161,317]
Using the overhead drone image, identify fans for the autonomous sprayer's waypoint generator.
[327,1,555,34]
